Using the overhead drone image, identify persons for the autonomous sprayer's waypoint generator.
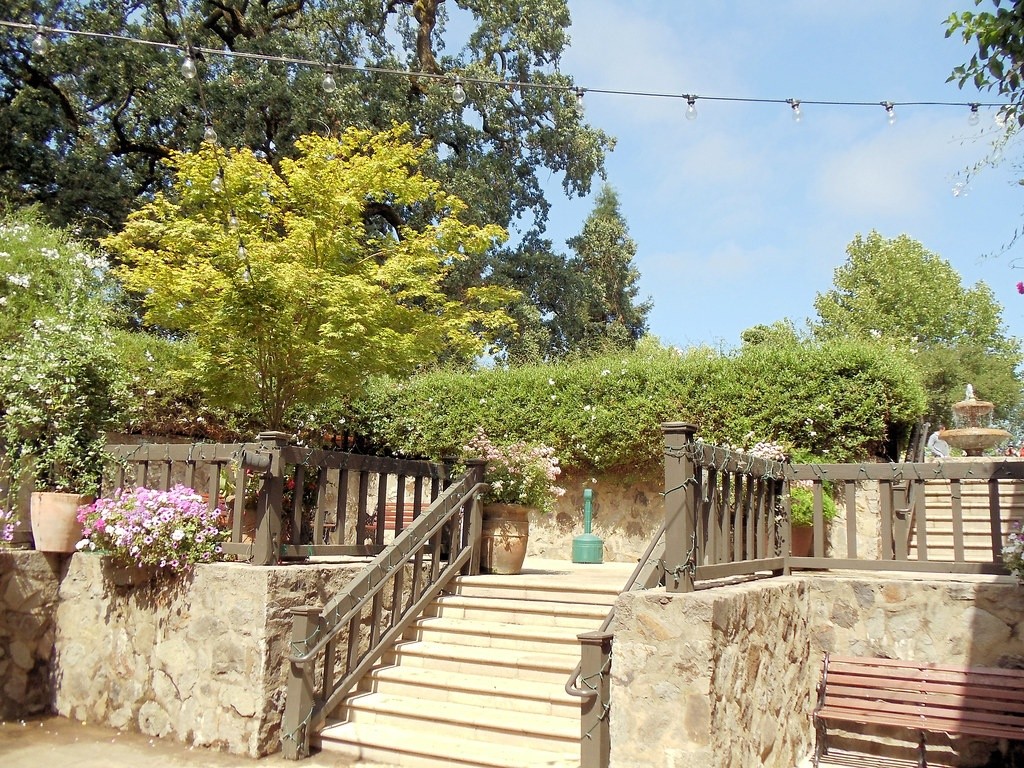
[927,424,950,457]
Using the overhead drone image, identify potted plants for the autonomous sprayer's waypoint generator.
[779,485,837,571]
[0,316,145,552]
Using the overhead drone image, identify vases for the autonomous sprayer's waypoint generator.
[482,502,529,574]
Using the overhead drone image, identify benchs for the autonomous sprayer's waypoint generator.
[807,650,1024,768]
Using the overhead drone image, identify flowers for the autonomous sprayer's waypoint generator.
[456,426,567,515]
[1000,520,1024,580]
[74,484,222,579]
